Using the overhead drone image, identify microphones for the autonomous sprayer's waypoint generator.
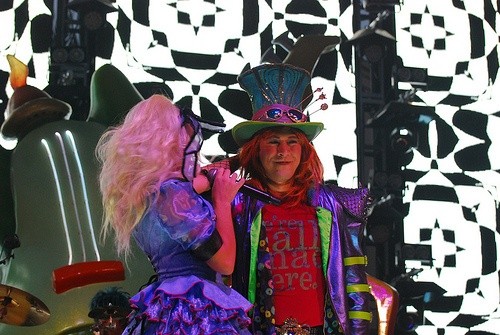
[206,168,282,207]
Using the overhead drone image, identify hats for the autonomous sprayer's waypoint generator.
[231,63,324,147]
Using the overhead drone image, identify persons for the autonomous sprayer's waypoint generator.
[94,94,256,335]
[200,62,374,334]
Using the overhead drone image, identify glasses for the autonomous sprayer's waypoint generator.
[255,107,308,123]
